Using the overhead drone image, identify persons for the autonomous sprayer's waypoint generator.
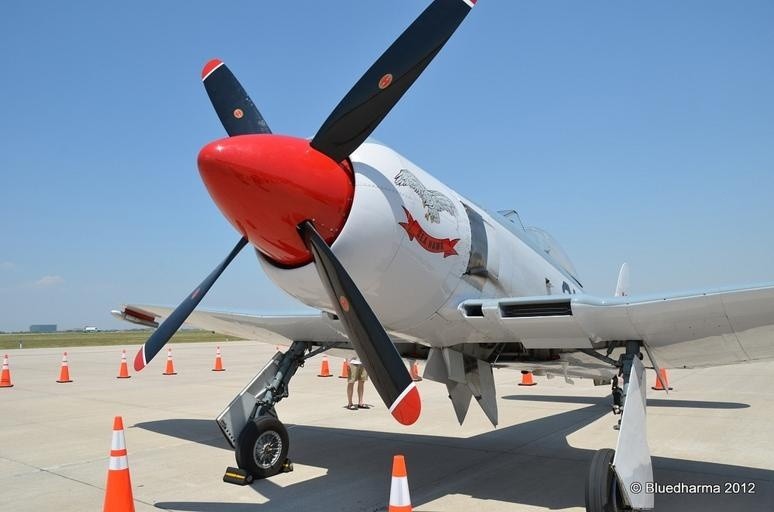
[345,348,370,410]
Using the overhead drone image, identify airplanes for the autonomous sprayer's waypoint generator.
[109,1,773,512]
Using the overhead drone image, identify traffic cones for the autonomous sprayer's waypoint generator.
[649,368,674,391]
[407,361,422,381]
[100,414,135,512]
[337,358,348,379]
[517,371,538,386]
[383,453,414,512]
[113,349,131,379]
[315,354,333,377]
[0,354,14,388]
[55,351,73,383]
[162,346,178,375]
[209,345,225,371]
